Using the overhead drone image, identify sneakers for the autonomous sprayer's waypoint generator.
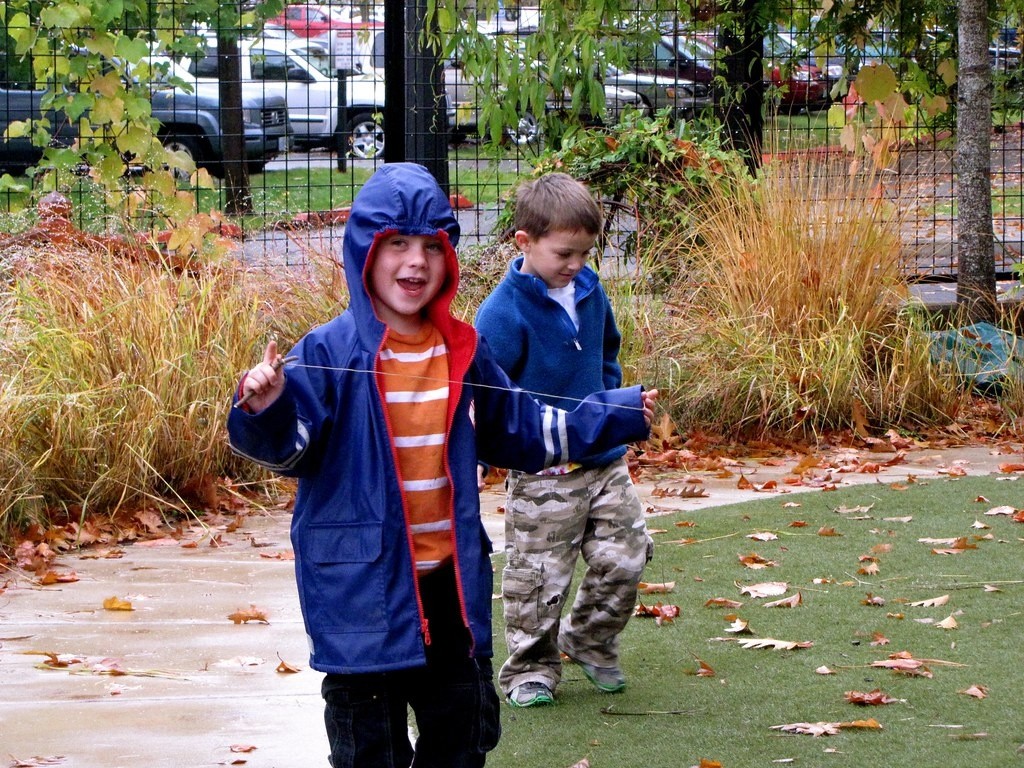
[559,643,627,692]
[509,682,555,708]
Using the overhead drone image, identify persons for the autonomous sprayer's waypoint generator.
[469,169,654,709]
[227,161,661,767]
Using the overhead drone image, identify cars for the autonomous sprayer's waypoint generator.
[0,37,296,183]
[158,0,1024,160]
